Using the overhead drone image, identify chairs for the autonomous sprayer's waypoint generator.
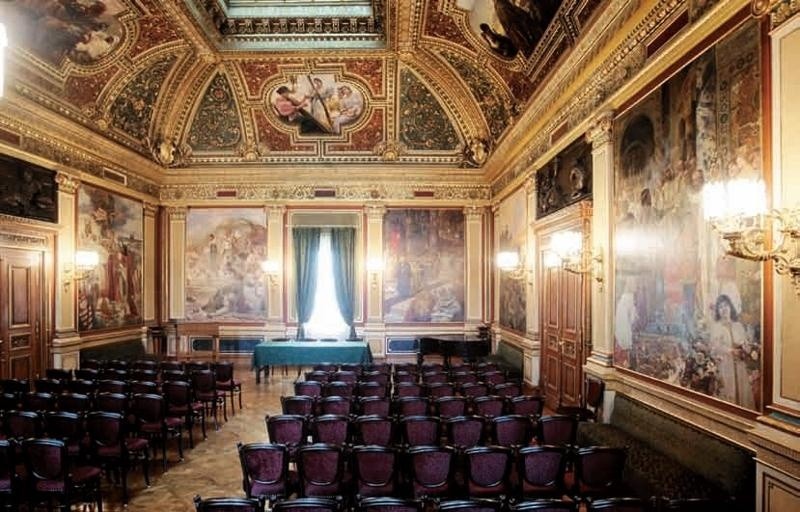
[0,360,242,512]
[193,361,709,511]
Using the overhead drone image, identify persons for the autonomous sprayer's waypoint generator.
[613,167,757,412]
[273,78,361,136]
[395,251,411,298]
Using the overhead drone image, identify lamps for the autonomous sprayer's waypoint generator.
[62,249,101,287]
[550,229,608,289]
[699,178,800,288]
[493,249,535,288]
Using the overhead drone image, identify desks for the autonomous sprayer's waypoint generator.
[174,320,222,360]
[250,341,375,385]
